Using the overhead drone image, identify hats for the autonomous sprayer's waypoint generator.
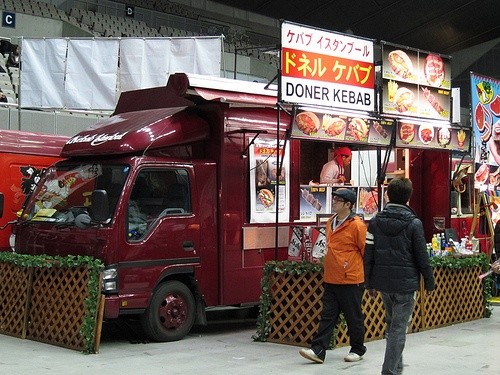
[333,189,356,204]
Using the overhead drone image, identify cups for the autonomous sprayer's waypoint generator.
[388,82,398,102]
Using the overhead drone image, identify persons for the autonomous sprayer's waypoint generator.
[299,190,368,363]
[320,146,352,183]
[363,177,434,375]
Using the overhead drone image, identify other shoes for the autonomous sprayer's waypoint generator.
[344,352,363,361]
[299,348,324,363]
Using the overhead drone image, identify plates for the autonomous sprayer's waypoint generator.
[437,128,452,148]
[419,123,434,145]
[399,124,416,145]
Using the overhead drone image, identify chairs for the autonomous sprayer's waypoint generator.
[159,182,189,211]
[0,0,280,117]
[107,182,122,212]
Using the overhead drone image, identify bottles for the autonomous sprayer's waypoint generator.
[425,232,480,257]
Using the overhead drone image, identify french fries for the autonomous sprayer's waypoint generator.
[457,130,466,142]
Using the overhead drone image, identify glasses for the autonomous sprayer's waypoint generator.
[334,198,345,203]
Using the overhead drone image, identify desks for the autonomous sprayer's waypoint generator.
[254,250,488,349]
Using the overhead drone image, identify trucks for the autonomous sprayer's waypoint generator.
[1,71,496,340]
